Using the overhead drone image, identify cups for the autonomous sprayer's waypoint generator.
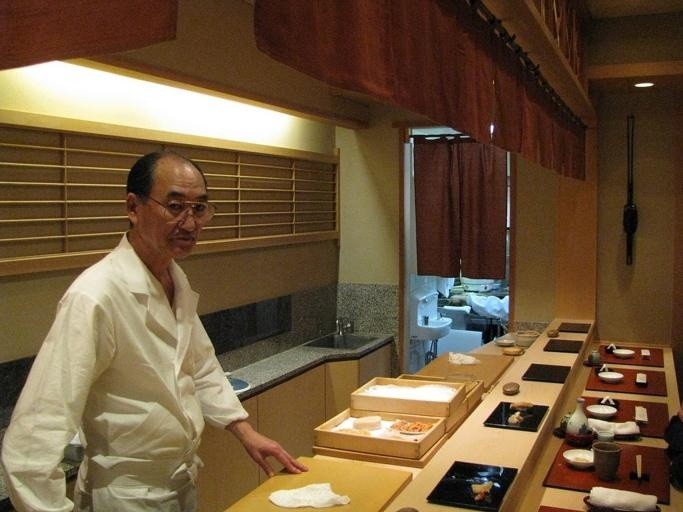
[592,441,623,480]
[597,431,613,442]
[565,429,593,446]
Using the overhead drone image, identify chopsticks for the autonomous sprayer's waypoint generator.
[606,343,616,349]
[599,364,608,371]
[635,454,641,478]
[601,395,615,405]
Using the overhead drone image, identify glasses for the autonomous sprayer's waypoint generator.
[136,190,215,226]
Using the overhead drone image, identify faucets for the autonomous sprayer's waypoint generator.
[336,318,352,336]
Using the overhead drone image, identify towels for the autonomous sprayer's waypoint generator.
[448,351,481,366]
[265,481,351,509]
[588,483,659,511]
[584,416,640,439]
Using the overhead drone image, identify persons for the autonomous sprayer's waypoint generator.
[0,150,309,512]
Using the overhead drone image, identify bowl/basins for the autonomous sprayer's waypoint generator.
[598,371,624,383]
[514,330,539,347]
[562,447,592,469]
[604,345,620,353]
[598,398,618,407]
[586,404,617,419]
[595,367,613,376]
[612,349,635,358]
[501,348,523,356]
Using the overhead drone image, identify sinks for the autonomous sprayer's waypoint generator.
[303,334,379,351]
[416,317,453,340]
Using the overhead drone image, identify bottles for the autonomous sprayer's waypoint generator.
[587,351,601,363]
[560,396,588,432]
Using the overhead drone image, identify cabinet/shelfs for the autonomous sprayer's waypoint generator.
[191,337,394,511]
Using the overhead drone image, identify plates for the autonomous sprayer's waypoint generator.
[493,339,515,347]
[635,406,648,422]
[635,373,646,383]
[582,494,661,511]
[553,427,565,437]
[582,360,603,366]
[641,349,650,359]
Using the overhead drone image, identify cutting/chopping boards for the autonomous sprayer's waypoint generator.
[311,409,469,469]
[413,351,514,392]
[224,455,413,512]
